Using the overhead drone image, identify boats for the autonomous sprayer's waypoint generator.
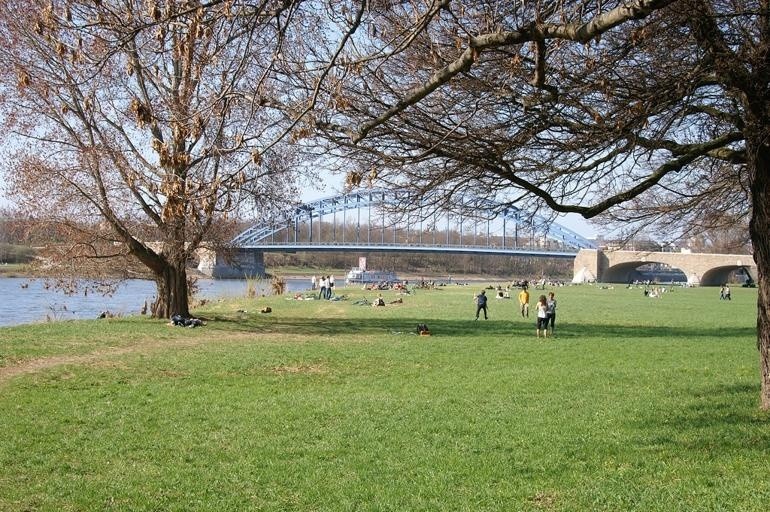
[344,266,403,287]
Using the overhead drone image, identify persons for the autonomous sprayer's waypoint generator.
[518,286,529,319]
[534,295,549,339]
[719,285,725,301]
[312,274,335,301]
[544,291,557,336]
[284,291,318,301]
[330,275,446,307]
[473,289,488,321]
[495,275,616,299]
[625,277,695,300]
[723,285,731,300]
